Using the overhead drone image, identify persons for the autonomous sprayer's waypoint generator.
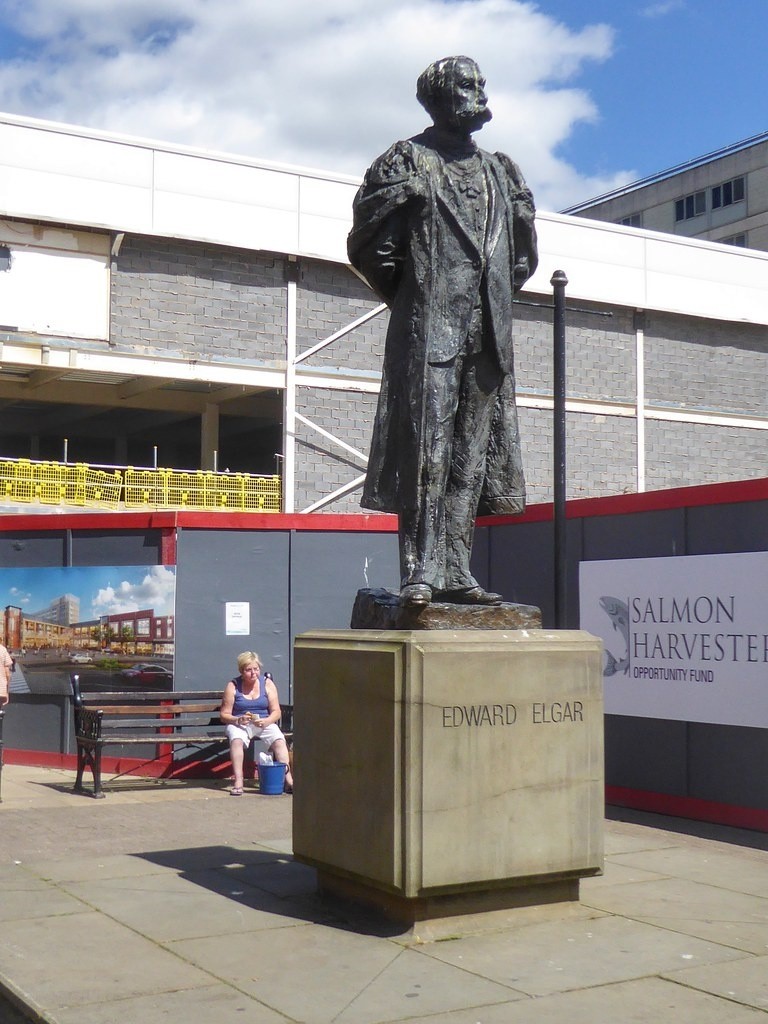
[220,651,293,795]
[21,644,135,660]
[0,644,13,706]
[344,54,540,606]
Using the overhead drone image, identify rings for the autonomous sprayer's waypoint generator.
[259,723,262,727]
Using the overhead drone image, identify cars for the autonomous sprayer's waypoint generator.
[120,664,172,686]
[68,654,93,664]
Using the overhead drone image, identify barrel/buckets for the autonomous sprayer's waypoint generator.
[257,762,288,795]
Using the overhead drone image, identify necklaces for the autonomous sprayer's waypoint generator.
[445,148,485,198]
[244,678,256,696]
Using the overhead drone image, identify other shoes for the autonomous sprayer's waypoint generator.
[230,787,244,795]
[286,784,293,794]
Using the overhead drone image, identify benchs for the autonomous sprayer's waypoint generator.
[73,674,294,799]
